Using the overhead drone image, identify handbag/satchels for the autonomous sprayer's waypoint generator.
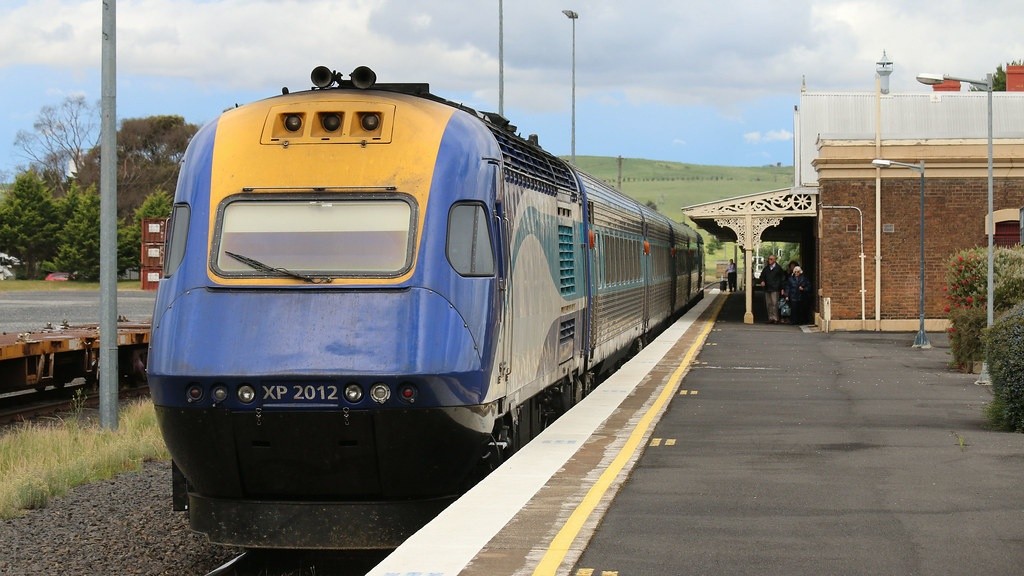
[781,300,791,315]
[778,294,785,309]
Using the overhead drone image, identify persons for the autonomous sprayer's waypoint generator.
[760,255,813,324]
[724,259,737,292]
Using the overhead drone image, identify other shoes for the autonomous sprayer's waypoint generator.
[769,316,779,323]
[781,319,791,323]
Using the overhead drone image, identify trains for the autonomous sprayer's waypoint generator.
[143,65,706,549]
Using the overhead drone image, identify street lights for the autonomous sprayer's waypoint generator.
[872,158,932,351]
[915,71,994,387]
[562,9,579,166]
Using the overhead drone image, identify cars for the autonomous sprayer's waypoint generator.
[44,271,78,281]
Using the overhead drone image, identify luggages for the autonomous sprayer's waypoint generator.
[720,274,727,292]
